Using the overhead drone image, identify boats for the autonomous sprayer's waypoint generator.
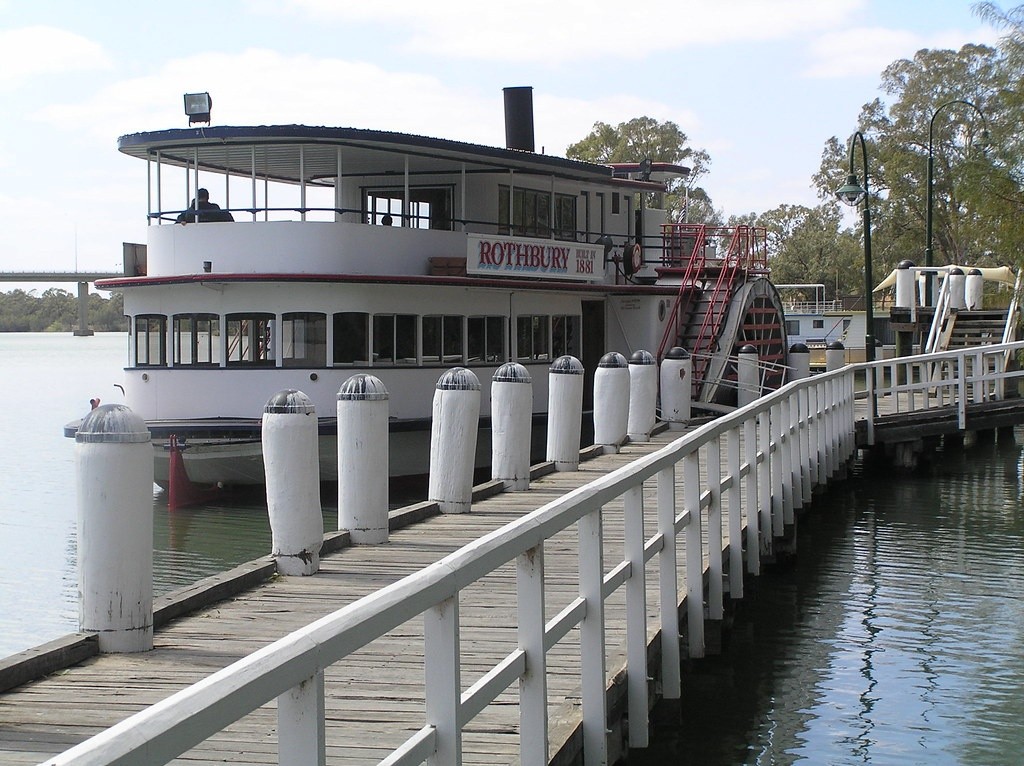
[58,85,791,516]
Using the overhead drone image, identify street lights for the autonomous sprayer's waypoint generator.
[834,131,882,417]
[917,100,995,370]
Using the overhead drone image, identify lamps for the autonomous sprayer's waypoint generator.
[640,159,652,169]
[184,92,212,127]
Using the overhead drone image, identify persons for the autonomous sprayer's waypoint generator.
[175,187,219,223]
[381,215,393,226]
